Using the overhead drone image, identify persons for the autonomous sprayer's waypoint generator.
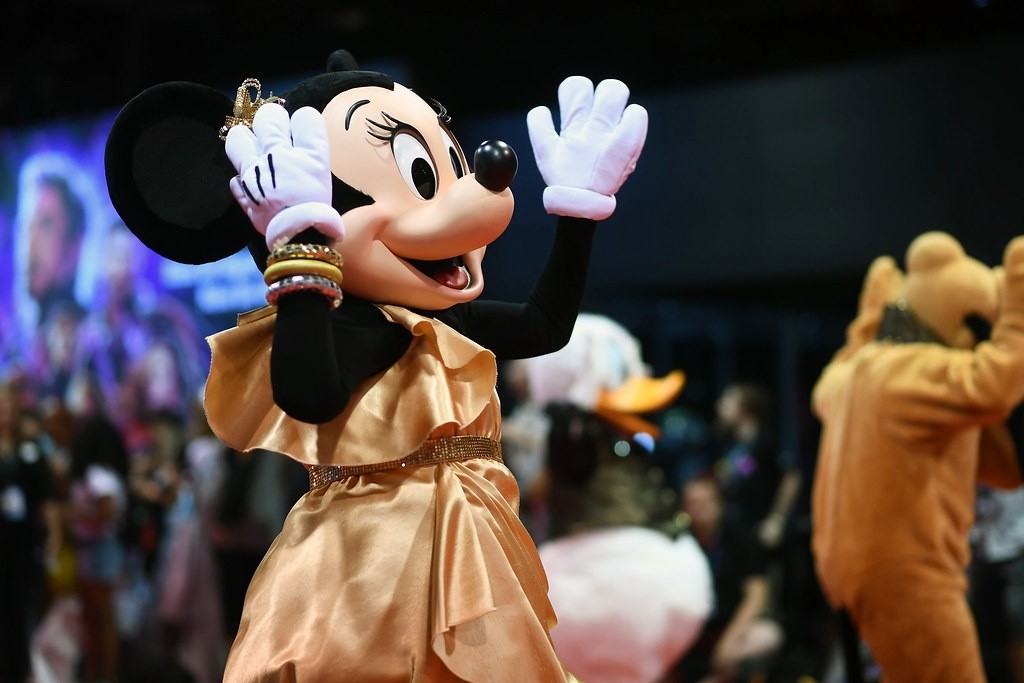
[24,176,208,406]
[659,361,863,683]
[0,366,309,683]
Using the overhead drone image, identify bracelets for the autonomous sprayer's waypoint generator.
[265,243,343,309]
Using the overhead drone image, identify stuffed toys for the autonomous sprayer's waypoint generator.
[104,50,648,683]
[810,231,1024,683]
[498,314,713,683]
